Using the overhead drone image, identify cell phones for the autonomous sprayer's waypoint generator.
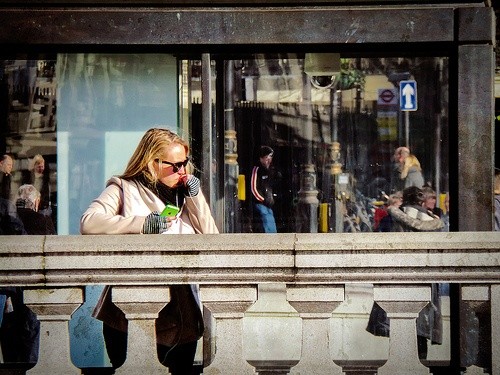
[160,205,180,218]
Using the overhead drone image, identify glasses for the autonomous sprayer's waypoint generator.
[154,156,190,173]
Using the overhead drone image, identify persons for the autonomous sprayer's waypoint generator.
[80,128,220,368]
[0,184,55,364]
[366,188,443,359]
[251,146,277,233]
[374,148,444,232]
[0,155,49,235]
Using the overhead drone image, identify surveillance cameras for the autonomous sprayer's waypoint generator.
[304,53,341,89]
